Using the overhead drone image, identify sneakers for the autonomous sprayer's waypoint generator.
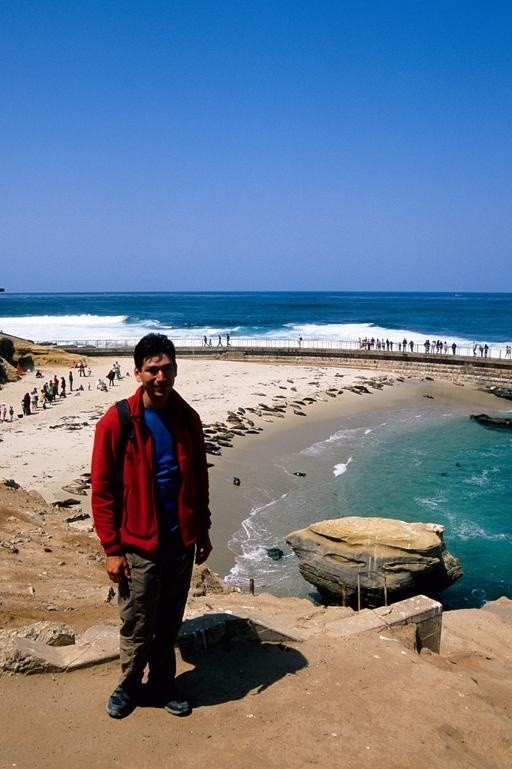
[164,691,191,715]
[106,684,140,718]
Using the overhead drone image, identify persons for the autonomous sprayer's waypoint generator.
[298,333,302,344]
[0,359,121,421]
[217,334,223,346]
[204,334,210,346]
[355,336,511,358]
[226,333,230,345]
[91,332,213,719]
